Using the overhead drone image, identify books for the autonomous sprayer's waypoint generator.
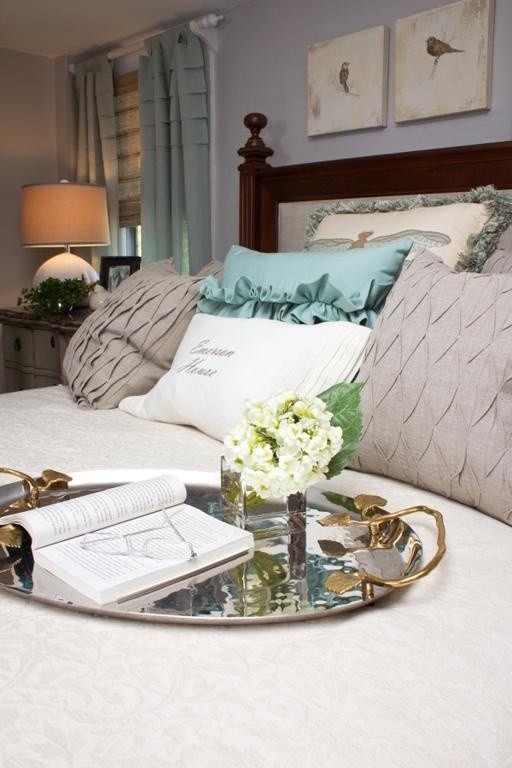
[0,472,258,606]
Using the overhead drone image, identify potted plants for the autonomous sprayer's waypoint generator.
[16,276,99,316]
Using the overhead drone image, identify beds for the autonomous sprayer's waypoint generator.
[2,112,510,768]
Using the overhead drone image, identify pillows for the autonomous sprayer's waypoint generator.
[59,184,510,527]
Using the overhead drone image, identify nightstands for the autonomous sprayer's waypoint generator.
[0,303,93,393]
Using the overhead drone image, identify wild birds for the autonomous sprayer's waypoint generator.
[423,36,469,65]
[339,61,352,94]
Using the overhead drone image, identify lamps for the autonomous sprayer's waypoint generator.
[21,178,111,292]
